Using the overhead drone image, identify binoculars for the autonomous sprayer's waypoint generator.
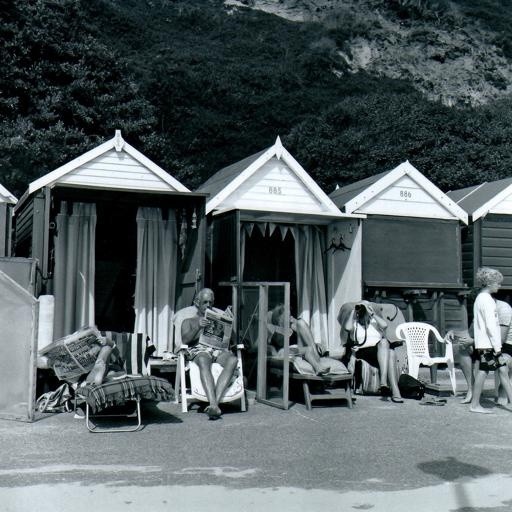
[355,304,366,311]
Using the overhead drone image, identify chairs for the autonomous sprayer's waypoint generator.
[172,304,248,414]
[71,329,149,433]
[395,321,458,396]
[254,310,355,410]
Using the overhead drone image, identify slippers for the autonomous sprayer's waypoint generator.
[378,385,392,397]
[419,395,447,406]
[391,394,403,402]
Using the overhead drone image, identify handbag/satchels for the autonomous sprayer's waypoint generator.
[399,373,425,400]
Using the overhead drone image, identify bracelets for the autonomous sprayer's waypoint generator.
[112,342,116,349]
[371,313,374,316]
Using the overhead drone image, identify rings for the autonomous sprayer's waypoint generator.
[101,342,102,345]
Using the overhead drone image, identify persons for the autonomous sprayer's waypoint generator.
[270,305,331,376]
[444,289,512,411]
[469,268,512,414]
[78,327,125,386]
[181,288,238,419]
[344,300,403,402]
